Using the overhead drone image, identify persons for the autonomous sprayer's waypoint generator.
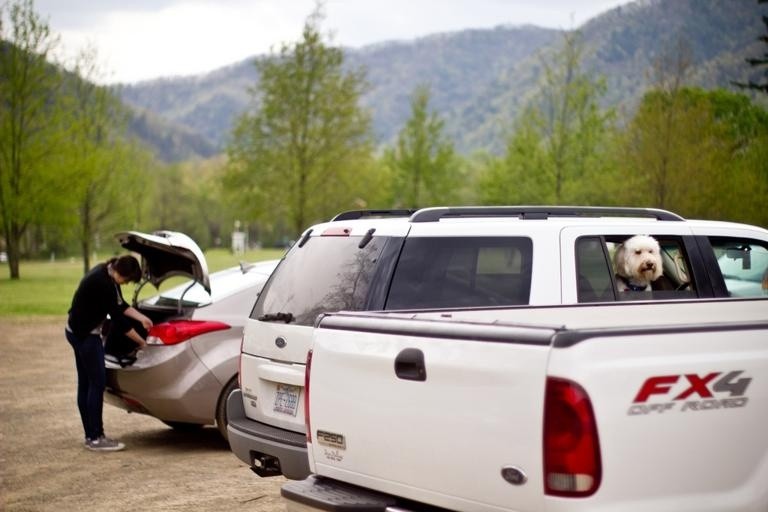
[65,256,153,452]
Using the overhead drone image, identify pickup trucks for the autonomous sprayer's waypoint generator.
[276,293,766,512]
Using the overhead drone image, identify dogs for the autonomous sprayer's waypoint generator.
[600,231,664,301]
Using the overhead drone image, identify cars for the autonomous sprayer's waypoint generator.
[97,225,281,451]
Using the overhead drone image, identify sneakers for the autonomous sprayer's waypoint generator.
[89,437,126,452]
[85,438,90,448]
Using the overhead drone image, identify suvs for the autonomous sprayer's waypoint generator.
[217,202,766,485]
